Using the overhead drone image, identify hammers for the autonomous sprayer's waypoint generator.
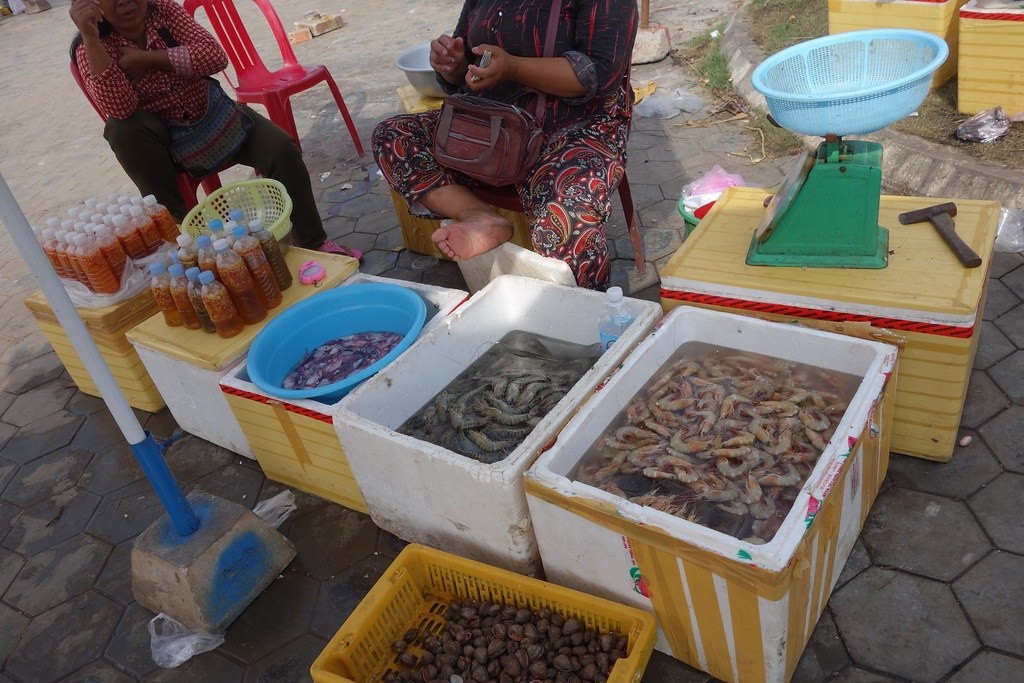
[898,201,983,269]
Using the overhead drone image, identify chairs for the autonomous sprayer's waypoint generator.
[182,0,365,177]
[467,51,648,278]
[69,60,239,217]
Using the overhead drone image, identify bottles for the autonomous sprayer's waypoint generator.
[150,209,293,337]
[598,286,635,353]
[41,192,181,294]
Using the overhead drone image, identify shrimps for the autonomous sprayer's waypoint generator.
[397,329,855,547]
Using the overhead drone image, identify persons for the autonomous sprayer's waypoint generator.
[371,0,640,293]
[68,0,366,261]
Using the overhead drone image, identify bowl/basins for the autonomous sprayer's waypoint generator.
[246,282,427,400]
[394,40,449,98]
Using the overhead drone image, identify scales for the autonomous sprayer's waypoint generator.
[745,28,950,271]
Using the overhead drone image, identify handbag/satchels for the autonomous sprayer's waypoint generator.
[431,92,544,189]
[165,77,254,179]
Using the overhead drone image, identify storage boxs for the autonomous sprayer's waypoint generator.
[333,274,664,582]
[957,0,1024,122]
[396,84,445,114]
[828,0,968,88]
[125,245,361,461]
[523,305,899,682]
[24,224,215,413]
[220,274,469,515]
[660,187,1002,463]
[310,542,658,683]
[389,184,534,260]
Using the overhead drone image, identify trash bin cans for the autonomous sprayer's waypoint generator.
[677,163,747,243]
